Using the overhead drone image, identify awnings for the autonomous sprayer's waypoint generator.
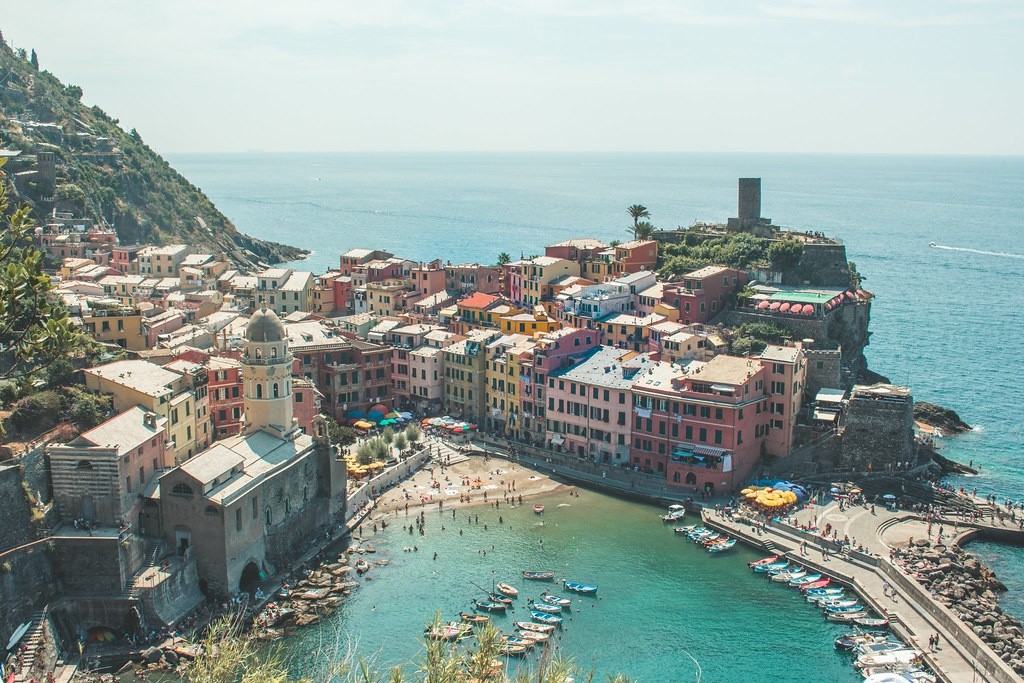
[551,438,564,445]
[674,442,723,457]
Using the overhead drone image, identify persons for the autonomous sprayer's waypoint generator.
[0,405,1024,683]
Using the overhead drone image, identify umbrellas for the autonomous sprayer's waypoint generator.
[736,477,806,508]
[337,404,476,432]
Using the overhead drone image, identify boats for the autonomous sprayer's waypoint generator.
[461,613,490,623]
[491,621,556,653]
[523,571,555,579]
[497,581,518,595]
[673,523,739,553]
[834,631,937,683]
[659,506,686,522]
[423,622,475,641]
[475,592,512,614]
[562,579,598,593]
[532,503,545,513]
[530,594,571,623]
[746,554,890,626]
